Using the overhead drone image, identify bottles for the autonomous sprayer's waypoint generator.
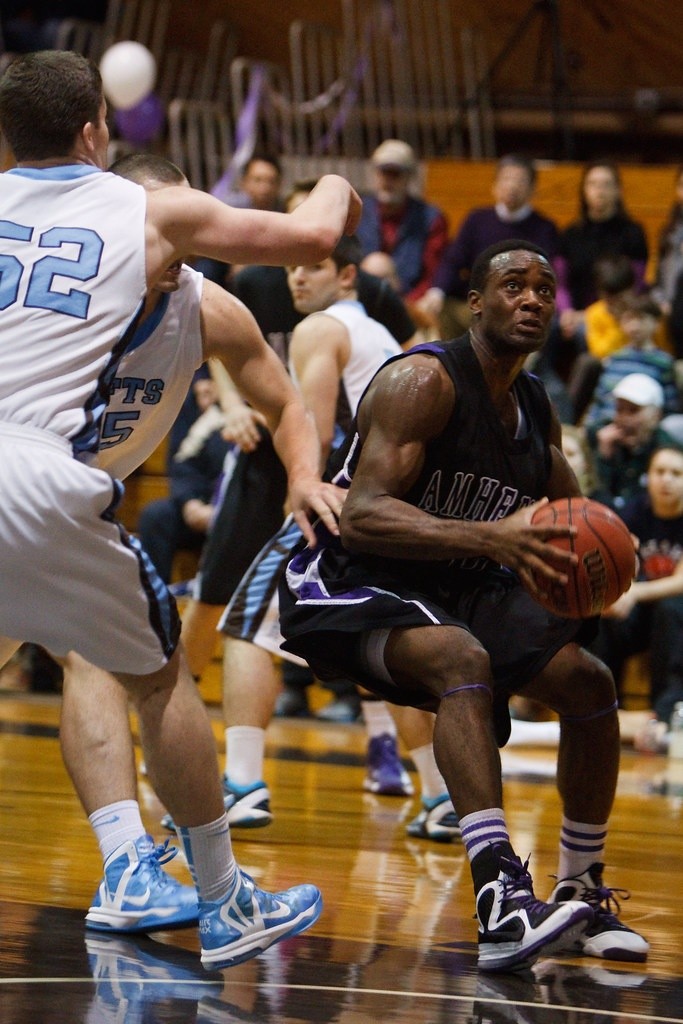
[667,702,683,813]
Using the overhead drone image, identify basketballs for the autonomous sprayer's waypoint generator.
[519,498,635,618]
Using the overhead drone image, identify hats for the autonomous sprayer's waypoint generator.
[371,139,416,170]
[662,415,683,442]
[614,372,663,408]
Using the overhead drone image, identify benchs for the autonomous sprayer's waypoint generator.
[114,432,652,713]
[424,158,683,286]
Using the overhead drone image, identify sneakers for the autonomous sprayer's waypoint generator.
[193,869,323,973]
[369,797,402,830]
[476,847,591,972]
[361,759,414,795]
[84,934,225,1024]
[199,996,259,1024]
[546,862,652,960]
[161,783,272,829]
[171,835,270,885]
[83,831,202,932]
[402,791,466,840]
[405,840,465,888]
[472,978,550,1024]
[533,961,647,1018]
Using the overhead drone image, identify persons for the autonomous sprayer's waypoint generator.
[160,234,463,839]
[0,48,364,969]
[139,136,683,785]
[0,152,350,932]
[278,246,649,967]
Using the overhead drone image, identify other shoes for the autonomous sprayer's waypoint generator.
[319,697,361,721]
[275,690,308,717]
